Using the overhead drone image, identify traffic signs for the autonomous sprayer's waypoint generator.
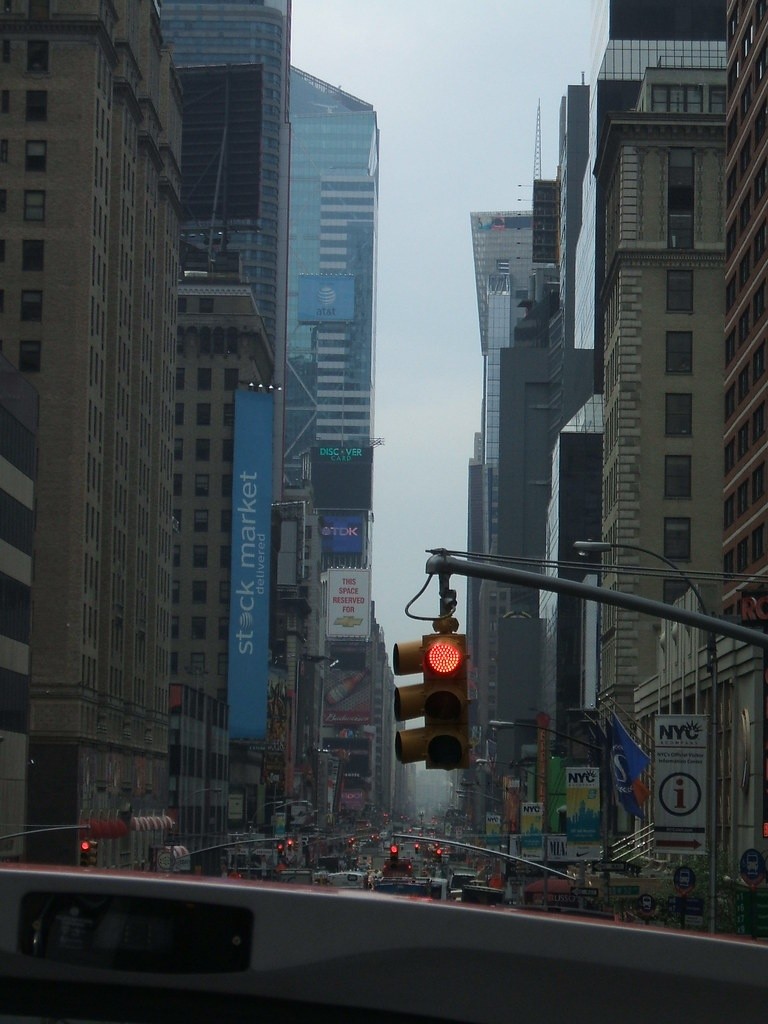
[653,712,707,856]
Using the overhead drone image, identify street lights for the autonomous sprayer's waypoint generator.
[191,787,224,853]
[573,540,718,936]
[269,800,312,881]
[475,759,548,912]
[454,789,510,885]
[247,801,283,880]
[488,721,608,860]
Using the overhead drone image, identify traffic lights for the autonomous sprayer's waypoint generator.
[88,841,98,866]
[389,844,398,869]
[81,841,90,867]
[278,843,283,858]
[392,639,427,765]
[419,632,472,768]
[436,849,442,863]
[287,841,292,851]
